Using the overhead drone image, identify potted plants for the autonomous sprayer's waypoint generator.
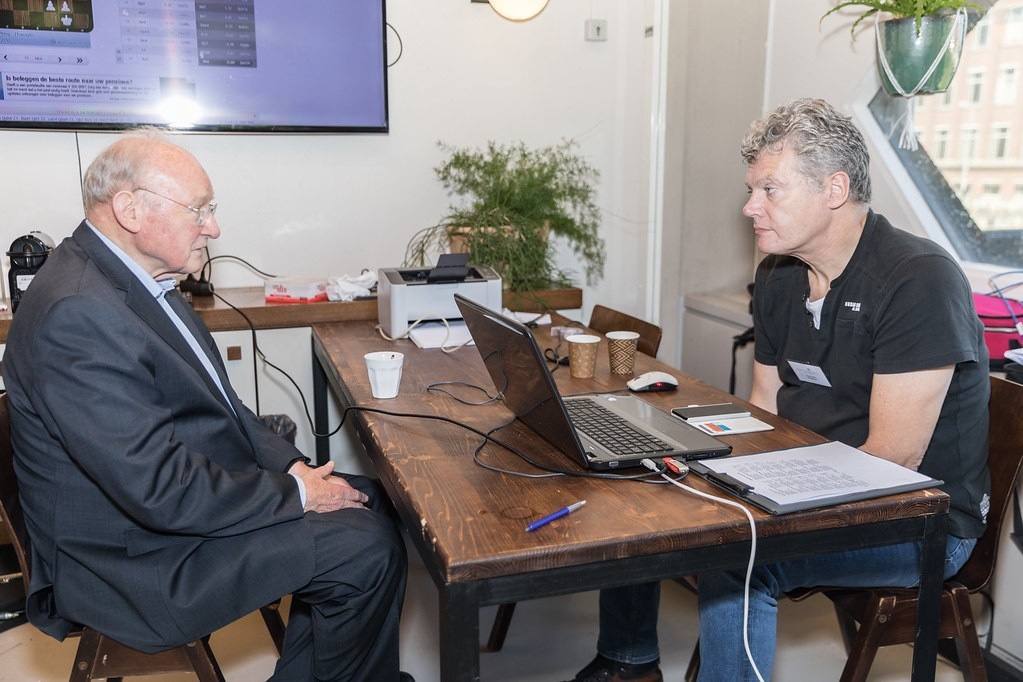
[400,139,610,296]
[820,1,981,94]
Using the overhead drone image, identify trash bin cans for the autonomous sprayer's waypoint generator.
[255,414,297,445]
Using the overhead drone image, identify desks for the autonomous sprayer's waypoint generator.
[311,316,950,682]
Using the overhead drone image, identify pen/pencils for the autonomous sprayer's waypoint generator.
[525,498,587,531]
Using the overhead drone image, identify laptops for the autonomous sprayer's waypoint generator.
[453,293,733,471]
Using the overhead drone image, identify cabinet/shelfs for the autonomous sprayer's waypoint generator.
[0,280,584,350]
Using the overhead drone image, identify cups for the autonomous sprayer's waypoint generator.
[564,334,601,378]
[363,351,404,399]
[606,331,640,376]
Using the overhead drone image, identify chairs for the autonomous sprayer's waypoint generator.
[486,302,662,653]
[682,372,1023,682]
[0,398,285,682]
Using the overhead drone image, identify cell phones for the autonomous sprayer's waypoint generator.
[670,402,751,422]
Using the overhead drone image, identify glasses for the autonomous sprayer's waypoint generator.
[131,188,218,227]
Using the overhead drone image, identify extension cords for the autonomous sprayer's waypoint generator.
[179,280,214,296]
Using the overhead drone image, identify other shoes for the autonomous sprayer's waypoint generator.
[399,671,415,682]
[566,654,663,682]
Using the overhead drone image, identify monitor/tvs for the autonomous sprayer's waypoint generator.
[0,0,390,134]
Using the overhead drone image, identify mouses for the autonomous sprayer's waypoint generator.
[626,371,678,393]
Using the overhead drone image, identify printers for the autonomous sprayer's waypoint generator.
[378,253,503,348]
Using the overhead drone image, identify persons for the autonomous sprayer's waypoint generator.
[573,98,991,682]
[0,135,409,682]
